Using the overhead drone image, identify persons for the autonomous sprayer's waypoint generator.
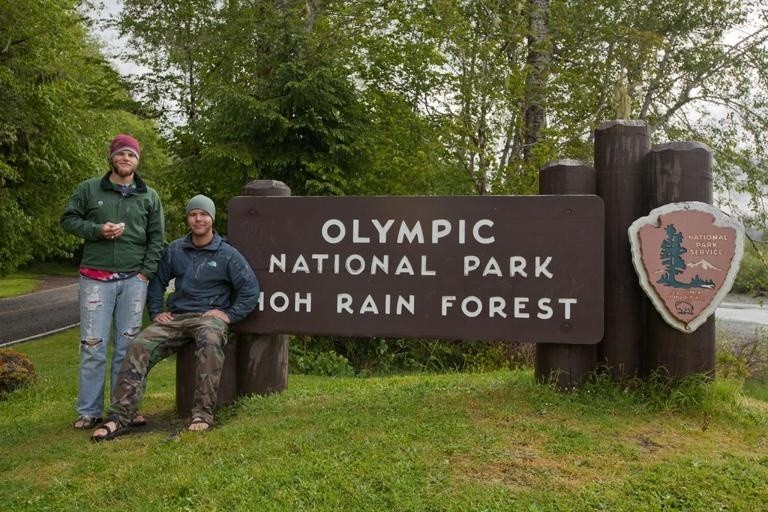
[91,193,260,441]
[60,134,165,430]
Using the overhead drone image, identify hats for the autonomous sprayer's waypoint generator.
[187,195,215,222]
[109,134,141,160]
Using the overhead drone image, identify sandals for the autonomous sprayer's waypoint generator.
[188,416,213,434]
[74,413,147,443]
